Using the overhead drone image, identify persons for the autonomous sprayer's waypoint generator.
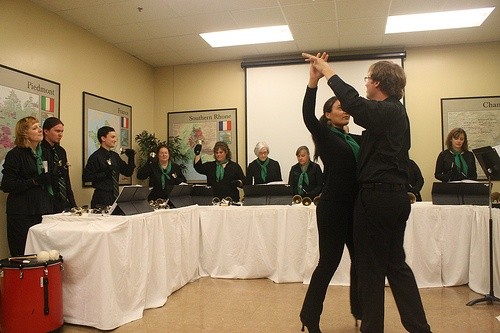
[193,141,246,202]
[138,145,187,201]
[302,52,431,333]
[82,127,137,209]
[246,142,283,186]
[0,116,58,257]
[288,145,323,201]
[435,128,477,182]
[409,158,425,202]
[42,117,77,214]
[299,52,368,333]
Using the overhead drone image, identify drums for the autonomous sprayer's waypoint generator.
[0,253,66,333]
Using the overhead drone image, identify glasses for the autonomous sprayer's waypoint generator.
[363,76,376,82]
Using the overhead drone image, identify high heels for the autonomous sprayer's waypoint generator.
[300,314,323,333]
[351,312,363,327]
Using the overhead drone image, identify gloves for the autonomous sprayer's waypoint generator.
[232,180,240,190]
[38,172,50,184]
[106,166,115,178]
[194,144,202,155]
[126,148,136,158]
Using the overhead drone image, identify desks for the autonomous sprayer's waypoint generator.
[24,197,500,331]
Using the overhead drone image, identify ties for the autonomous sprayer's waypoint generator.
[109,152,119,198]
[215,156,230,183]
[158,161,172,190]
[257,157,269,182]
[451,148,469,174]
[52,147,67,204]
[330,127,360,161]
[297,161,310,195]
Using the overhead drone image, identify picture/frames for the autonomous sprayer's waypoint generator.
[81,91,134,188]
[167,107,238,184]
[440,95,500,181]
[0,64,61,190]
